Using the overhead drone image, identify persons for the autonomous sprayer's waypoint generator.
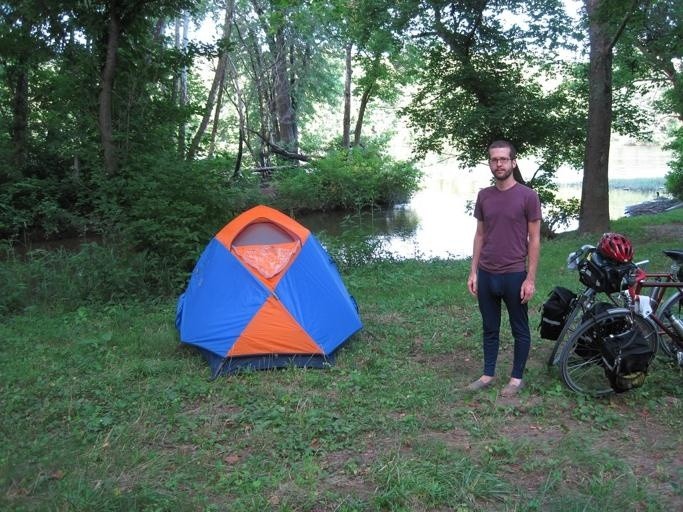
[467,141,541,397]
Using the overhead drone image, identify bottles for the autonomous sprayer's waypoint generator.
[666,312,683,340]
[649,276,662,304]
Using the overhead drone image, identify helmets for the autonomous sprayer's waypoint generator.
[596,233,634,263]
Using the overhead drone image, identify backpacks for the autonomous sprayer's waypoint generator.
[538,287,576,341]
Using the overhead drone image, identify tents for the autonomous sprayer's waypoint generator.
[177,204,363,376]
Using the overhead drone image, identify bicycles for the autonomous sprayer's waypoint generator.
[548,242,683,399]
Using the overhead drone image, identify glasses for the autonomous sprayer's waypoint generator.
[489,159,512,164]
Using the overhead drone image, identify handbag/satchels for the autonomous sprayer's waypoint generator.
[578,248,637,293]
[573,302,653,393]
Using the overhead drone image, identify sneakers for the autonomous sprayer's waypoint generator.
[501,381,524,397]
[469,376,495,390]
